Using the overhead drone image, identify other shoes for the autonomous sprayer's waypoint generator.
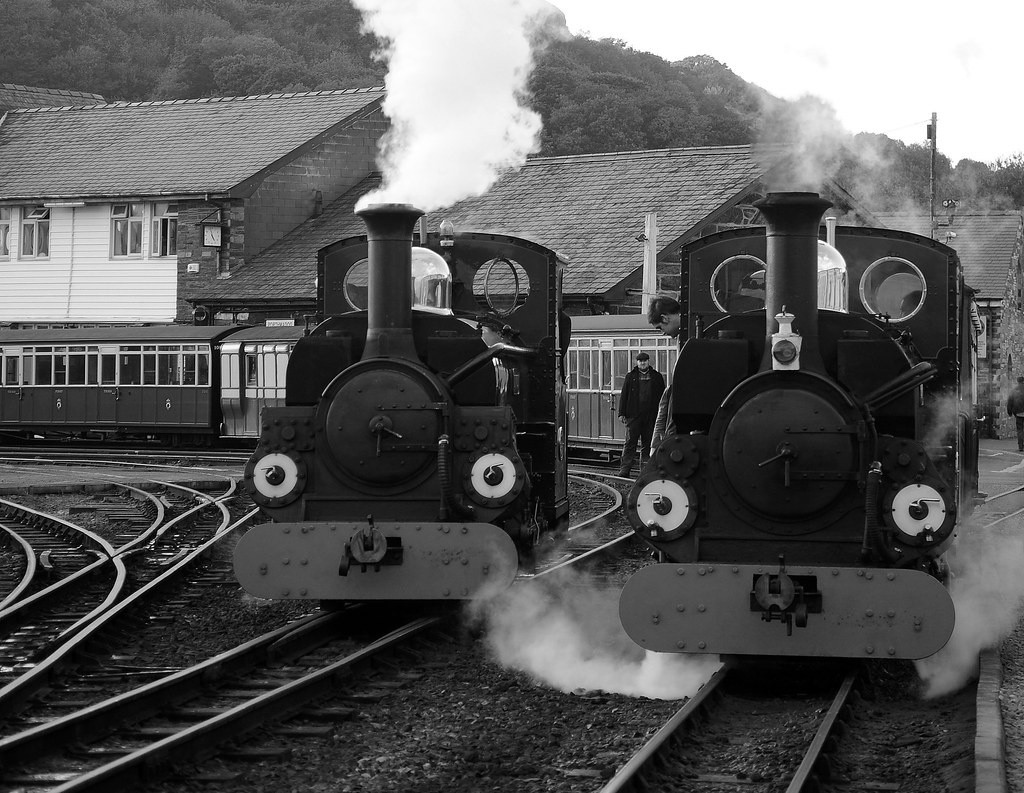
[619,469,630,478]
[1019,449,1023,451]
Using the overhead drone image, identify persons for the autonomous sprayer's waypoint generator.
[1007,377,1024,452]
[649,383,677,458]
[647,296,681,339]
[618,353,665,478]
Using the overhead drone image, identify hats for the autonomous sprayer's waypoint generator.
[1017,377,1024,382]
[636,353,649,360]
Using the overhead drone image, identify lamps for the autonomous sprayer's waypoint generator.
[44,202,85,208]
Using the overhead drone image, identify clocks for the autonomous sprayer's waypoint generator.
[200,225,223,247]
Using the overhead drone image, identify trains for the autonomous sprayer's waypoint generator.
[568,191,985,660]
[1,204,567,601]
